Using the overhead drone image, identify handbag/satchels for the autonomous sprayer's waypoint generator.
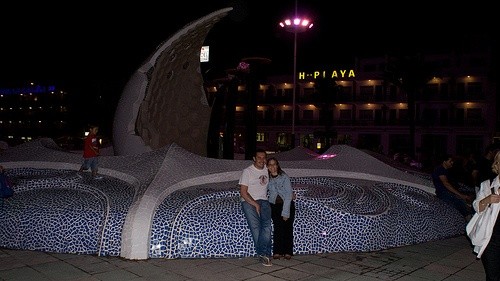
[465,179,494,247]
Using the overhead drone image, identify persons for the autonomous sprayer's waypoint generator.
[466,150,500,281]
[238,149,296,267]
[468,147,497,191]
[264,157,296,260]
[77,123,105,180]
[432,155,474,223]
[393,151,418,170]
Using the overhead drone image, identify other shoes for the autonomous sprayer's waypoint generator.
[273,253,291,261]
[259,256,273,267]
[91,175,104,180]
[76,172,83,178]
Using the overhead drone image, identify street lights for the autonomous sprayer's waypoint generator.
[279,15,314,150]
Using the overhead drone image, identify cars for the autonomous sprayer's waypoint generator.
[410,160,422,171]
